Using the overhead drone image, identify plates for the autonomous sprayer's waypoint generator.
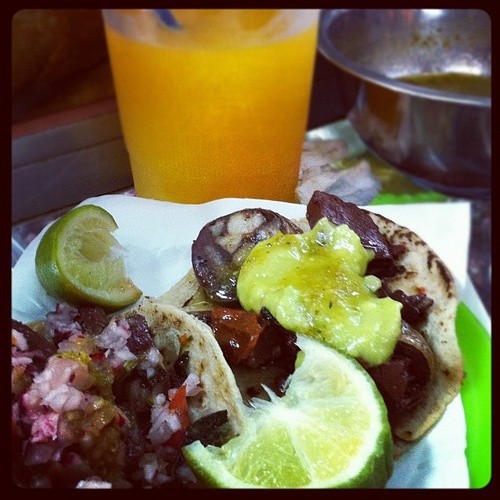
[13,194,492,490]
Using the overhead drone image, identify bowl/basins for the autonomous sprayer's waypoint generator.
[316,11,495,196]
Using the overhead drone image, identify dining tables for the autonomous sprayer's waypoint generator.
[8,117,495,490]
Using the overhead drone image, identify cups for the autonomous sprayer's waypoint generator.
[101,8,324,204]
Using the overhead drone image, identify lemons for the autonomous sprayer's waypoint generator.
[182,331,394,488]
[35,205,143,313]
